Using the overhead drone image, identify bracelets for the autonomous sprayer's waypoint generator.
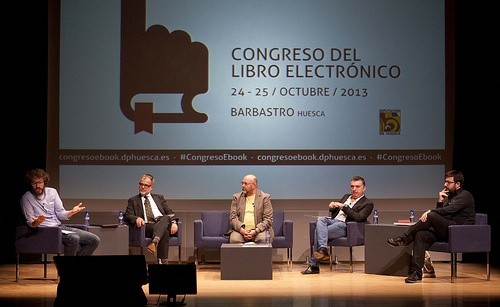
[172,221,177,223]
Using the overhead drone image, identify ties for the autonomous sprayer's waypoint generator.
[144,196,157,222]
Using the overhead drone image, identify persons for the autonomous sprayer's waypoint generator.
[387,170,475,283]
[228,174,274,244]
[123,174,180,264]
[21,169,101,257]
[301,176,374,274]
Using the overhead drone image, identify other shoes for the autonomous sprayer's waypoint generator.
[313,247,330,261]
[386,236,410,248]
[422,266,436,279]
[404,271,423,283]
[158,256,168,264]
[147,241,158,253]
[300,265,319,274]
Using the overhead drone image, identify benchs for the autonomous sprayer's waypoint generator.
[193,210,293,272]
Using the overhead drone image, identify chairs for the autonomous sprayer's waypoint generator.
[15,225,64,282]
[309,220,370,273]
[427,214,491,283]
[126,222,182,263]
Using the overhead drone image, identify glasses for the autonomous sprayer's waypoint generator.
[31,179,45,186]
[242,181,254,186]
[138,182,152,189]
[443,180,455,186]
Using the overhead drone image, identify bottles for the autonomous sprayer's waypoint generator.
[85,213,90,226]
[410,209,415,223]
[119,212,123,224]
[265,229,270,245]
[373,209,378,224]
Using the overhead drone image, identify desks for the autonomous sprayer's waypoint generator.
[364,222,417,276]
[64,223,129,255]
[221,243,272,280]
